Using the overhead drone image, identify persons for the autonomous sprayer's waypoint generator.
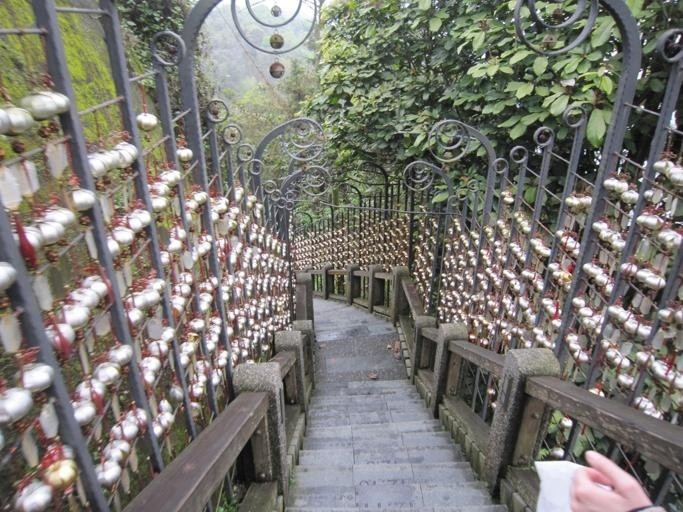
[569,451,669,512]
